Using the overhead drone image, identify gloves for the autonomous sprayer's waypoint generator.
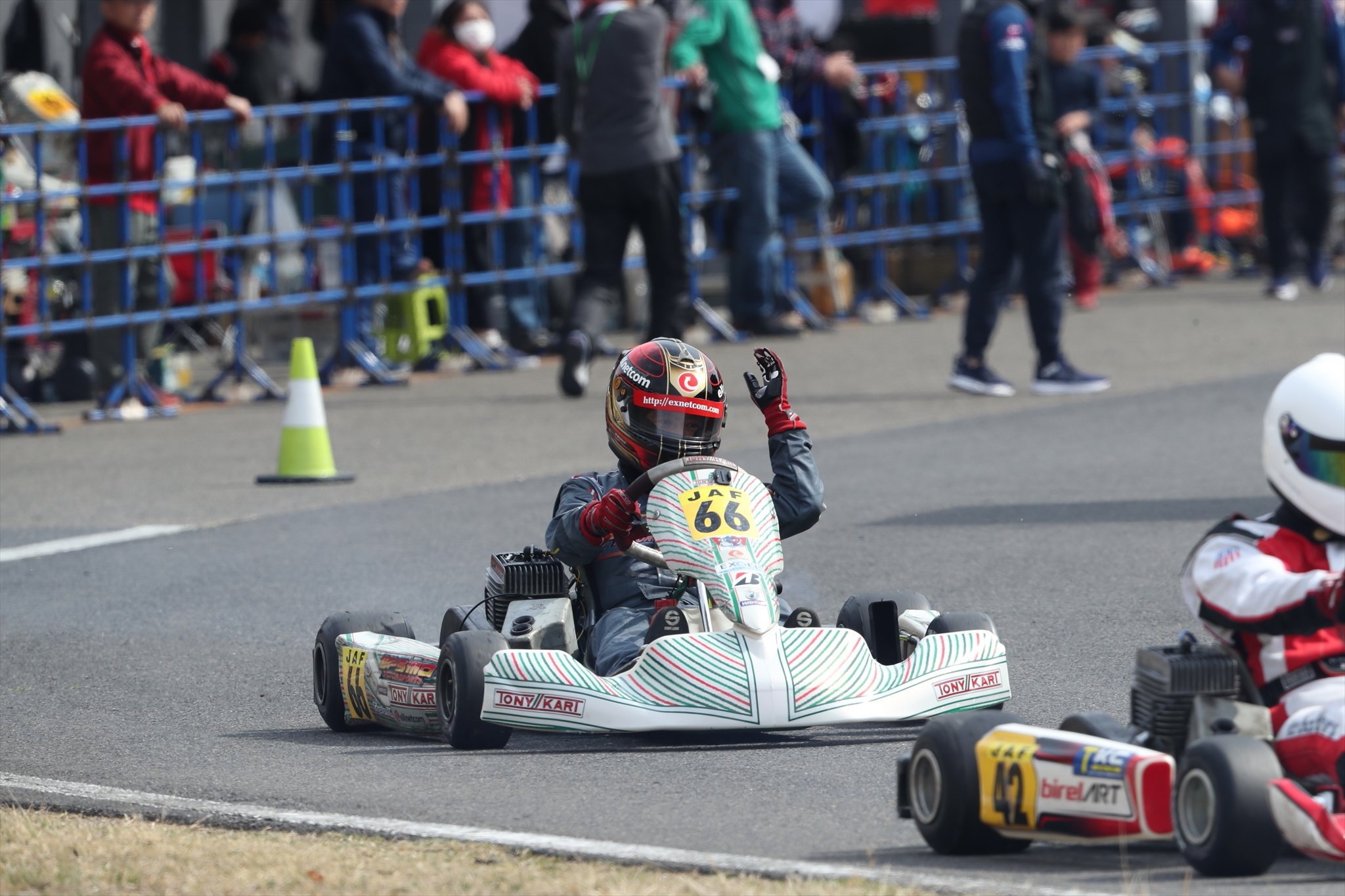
[585,487,644,536]
[743,346,806,434]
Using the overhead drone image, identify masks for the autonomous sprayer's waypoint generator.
[455,17,496,52]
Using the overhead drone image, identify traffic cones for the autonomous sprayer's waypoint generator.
[255,336,357,486]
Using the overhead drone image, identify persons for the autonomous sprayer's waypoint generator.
[560,0,692,396]
[214,0,1345,369]
[948,0,1111,394]
[1178,353,1345,815]
[546,338,827,676]
[76,0,249,419]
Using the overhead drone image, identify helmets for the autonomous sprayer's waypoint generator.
[1258,353,1345,537]
[605,336,729,473]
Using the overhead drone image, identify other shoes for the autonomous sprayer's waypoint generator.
[764,311,802,336]
[1030,353,1110,394]
[560,328,591,396]
[780,606,822,628]
[641,605,692,649]
[948,358,1014,399]
[1272,281,1298,301]
